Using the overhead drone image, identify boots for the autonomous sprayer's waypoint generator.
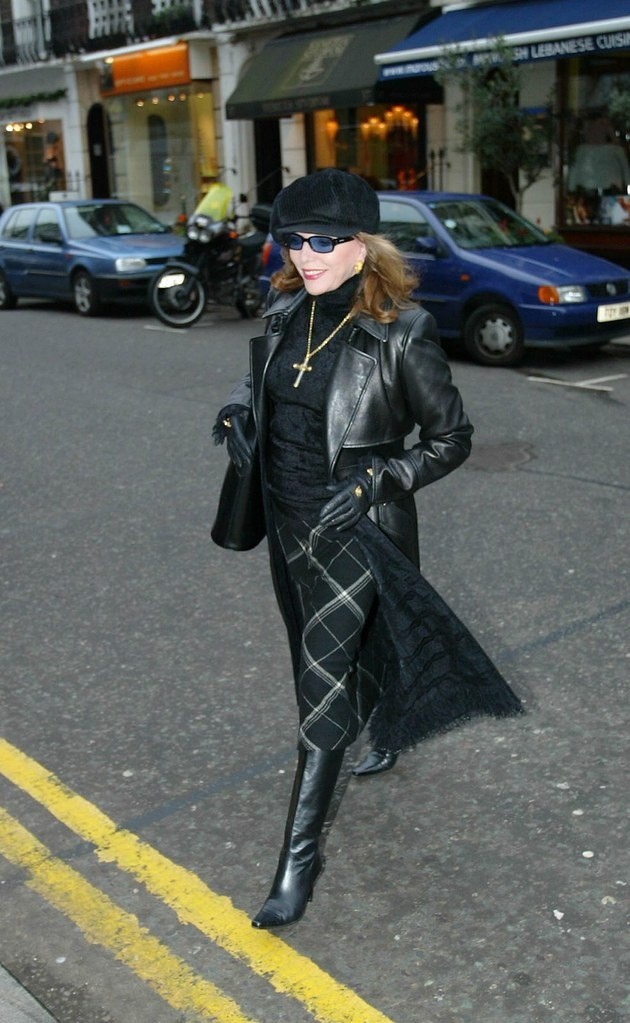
[252,749,344,928]
[351,747,402,775]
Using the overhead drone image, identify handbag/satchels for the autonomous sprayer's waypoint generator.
[210,458,267,550]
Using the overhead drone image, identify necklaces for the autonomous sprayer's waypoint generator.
[293,300,355,388]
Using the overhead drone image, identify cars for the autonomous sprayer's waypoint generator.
[0,198,187,317]
[259,191,630,368]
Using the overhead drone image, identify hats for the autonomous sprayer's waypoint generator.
[270,169,381,244]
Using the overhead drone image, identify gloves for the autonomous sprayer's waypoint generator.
[319,466,377,533]
[224,410,253,479]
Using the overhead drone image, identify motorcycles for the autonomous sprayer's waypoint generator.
[145,205,277,327]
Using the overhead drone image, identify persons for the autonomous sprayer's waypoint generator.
[216,168,521,928]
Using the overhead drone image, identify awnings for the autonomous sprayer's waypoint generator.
[225,11,443,118]
[371,0,630,81]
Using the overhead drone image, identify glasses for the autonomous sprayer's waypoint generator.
[285,231,355,254]
